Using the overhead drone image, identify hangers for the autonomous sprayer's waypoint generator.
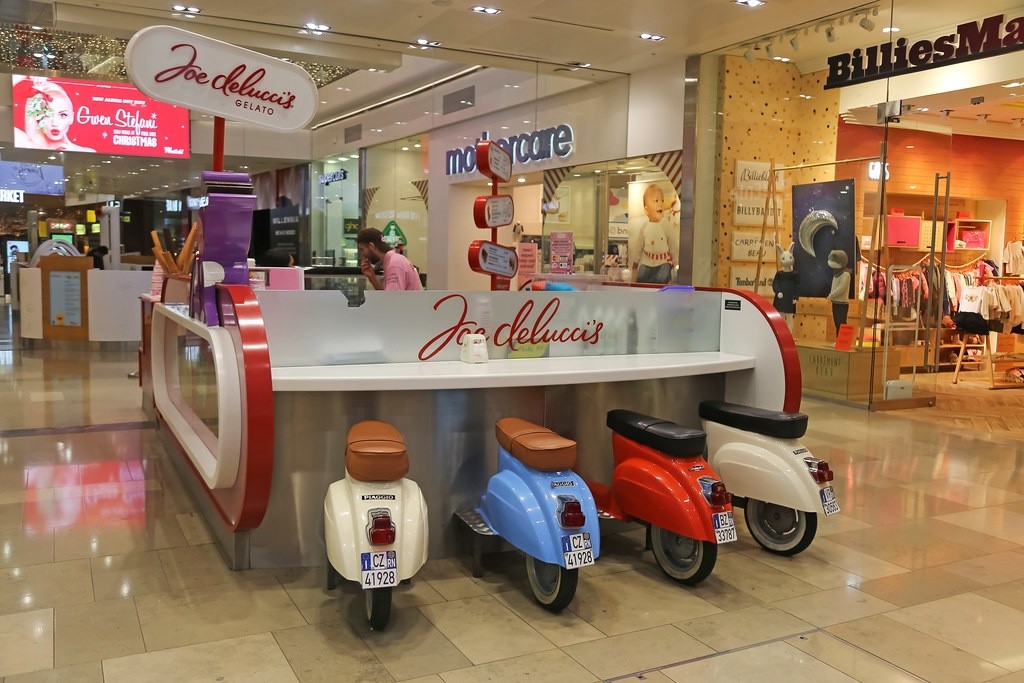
[967,275,1024,287]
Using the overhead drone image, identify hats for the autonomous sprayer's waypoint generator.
[358,228,392,252]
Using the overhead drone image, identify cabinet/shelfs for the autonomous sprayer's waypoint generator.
[861,216,992,252]
[888,328,986,373]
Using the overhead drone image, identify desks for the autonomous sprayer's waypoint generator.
[271,352,754,392]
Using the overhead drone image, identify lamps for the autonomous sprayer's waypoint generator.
[790,30,800,51]
[825,19,835,42]
[766,38,775,58]
[859,13,875,32]
[744,44,754,63]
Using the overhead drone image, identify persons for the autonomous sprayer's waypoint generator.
[632,185,677,284]
[357,228,425,290]
[826,250,850,337]
[87,246,109,270]
[25,82,96,152]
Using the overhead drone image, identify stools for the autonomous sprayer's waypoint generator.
[586,409,737,585]
[455,417,600,613]
[324,420,429,630]
[699,401,840,556]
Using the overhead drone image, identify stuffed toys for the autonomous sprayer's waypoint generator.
[772,242,806,313]
